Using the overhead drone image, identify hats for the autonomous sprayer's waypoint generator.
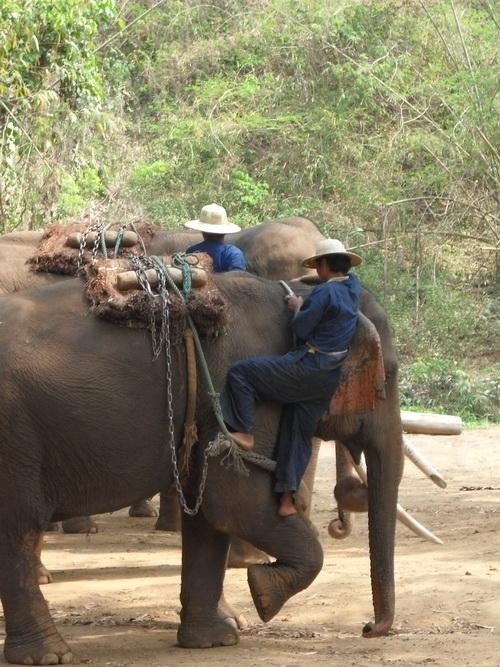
[184,204,242,235]
[301,238,363,268]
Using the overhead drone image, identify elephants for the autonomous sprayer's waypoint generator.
[0,264,402,662]
[5,214,368,571]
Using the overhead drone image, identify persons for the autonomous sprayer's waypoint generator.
[180,203,248,274]
[222,236,363,520]
